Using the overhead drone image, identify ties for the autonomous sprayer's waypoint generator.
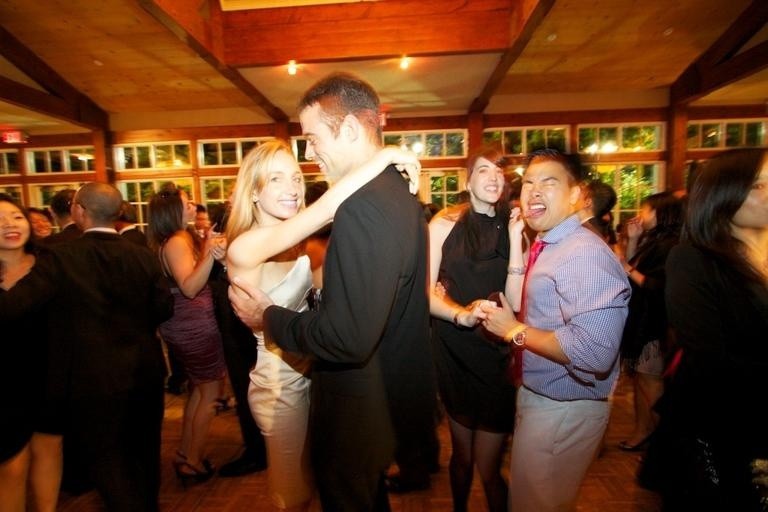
[512,241,549,390]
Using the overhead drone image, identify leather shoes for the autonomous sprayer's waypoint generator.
[217,445,267,480]
[618,434,647,453]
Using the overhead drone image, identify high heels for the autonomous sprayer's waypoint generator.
[171,450,215,488]
